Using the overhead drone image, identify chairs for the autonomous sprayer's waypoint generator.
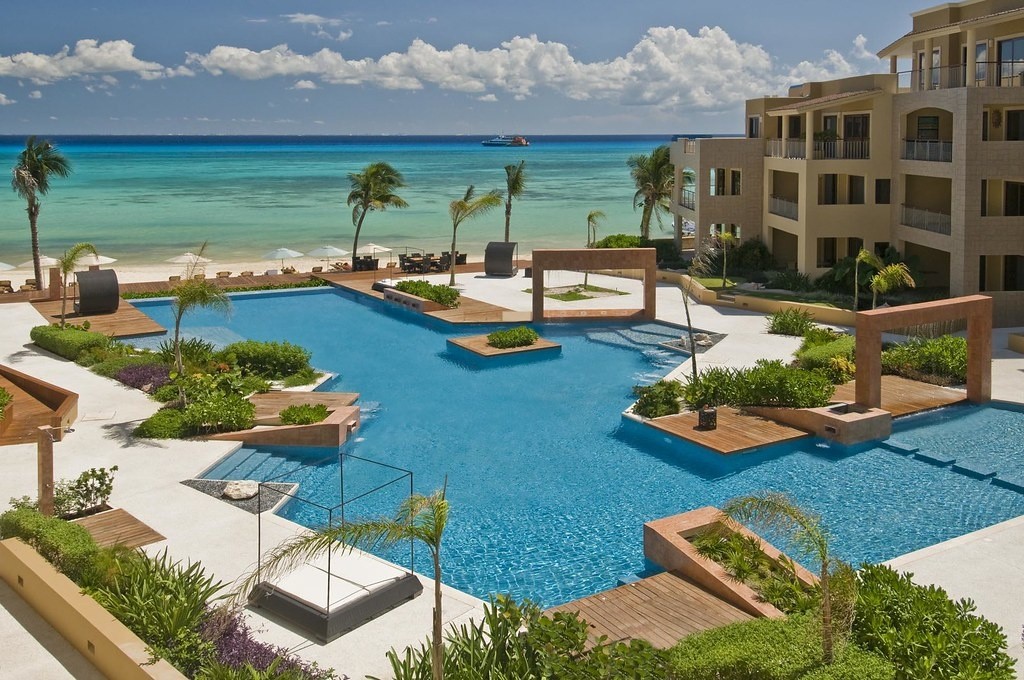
[398,251,467,274]
[351,256,379,271]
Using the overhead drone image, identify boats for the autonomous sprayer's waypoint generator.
[481,128,513,146]
[508,134,529,147]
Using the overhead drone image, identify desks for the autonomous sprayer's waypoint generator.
[408,256,443,274]
